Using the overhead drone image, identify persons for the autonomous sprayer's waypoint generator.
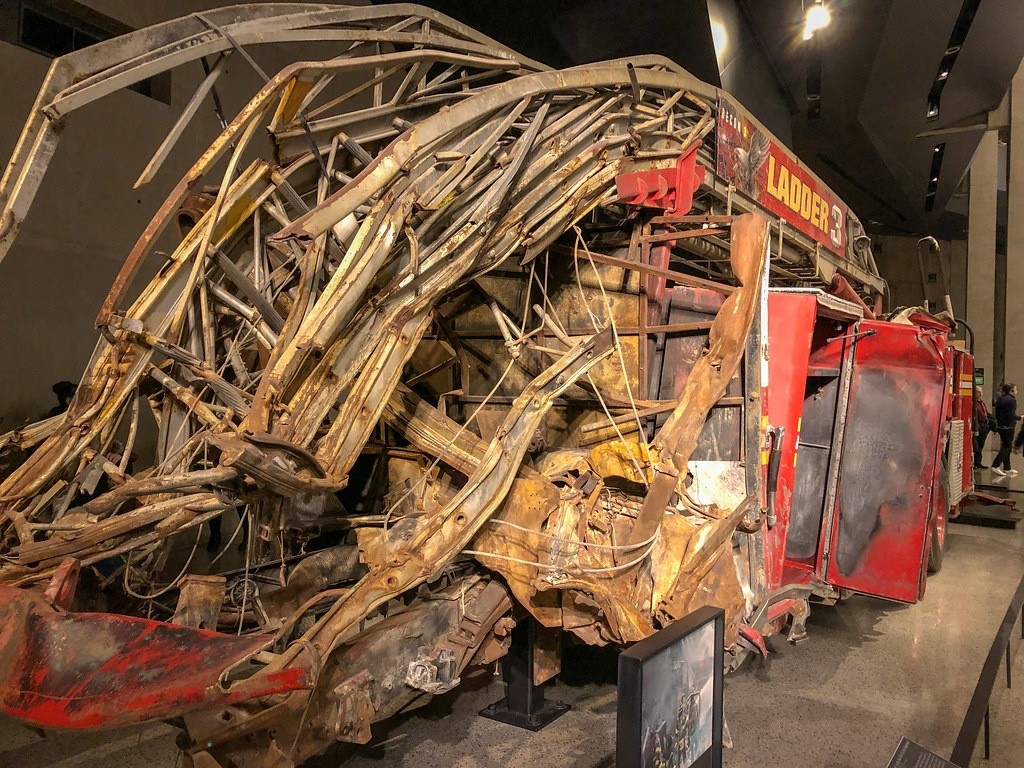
[976,386,990,469]
[990,383,1024,476]
[992,474,1018,499]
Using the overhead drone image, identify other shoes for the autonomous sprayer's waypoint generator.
[973,463,989,469]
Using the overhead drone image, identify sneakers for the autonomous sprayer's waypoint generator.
[1004,469,1018,474]
[990,466,1006,476]
